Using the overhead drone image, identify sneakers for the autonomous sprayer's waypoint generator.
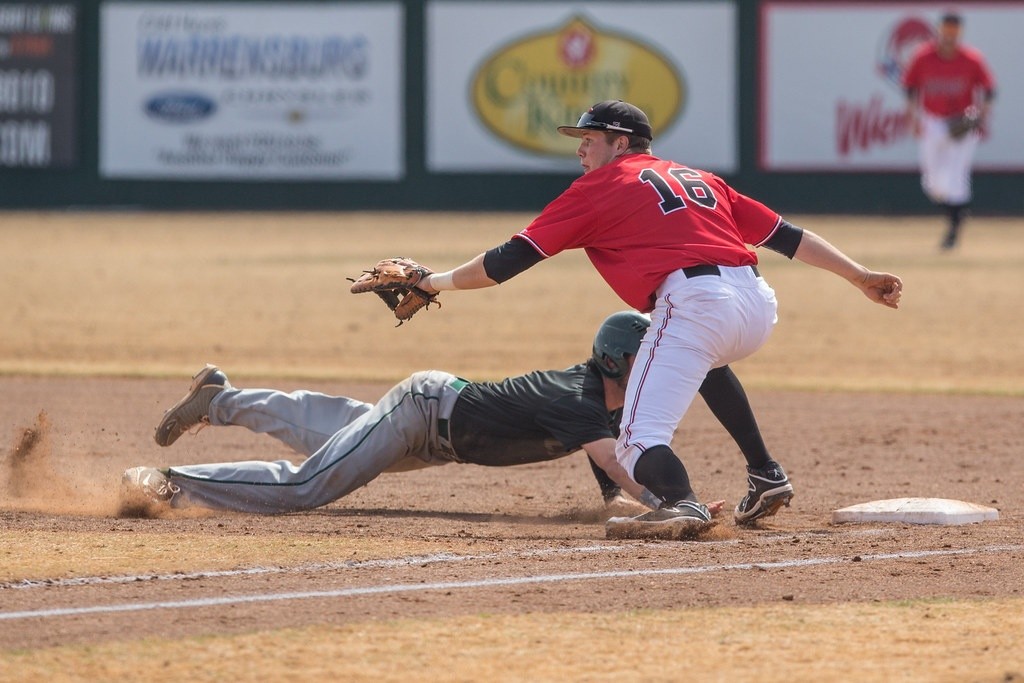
[153,364,229,448]
[605,500,713,534]
[734,463,793,525]
[121,465,181,519]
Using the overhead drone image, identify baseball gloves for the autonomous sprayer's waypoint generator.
[348,254,438,326]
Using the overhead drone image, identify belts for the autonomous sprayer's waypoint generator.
[437,378,470,457]
[682,265,761,278]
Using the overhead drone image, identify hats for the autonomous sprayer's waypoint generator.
[557,98,652,141]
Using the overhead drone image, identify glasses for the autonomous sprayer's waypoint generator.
[575,112,634,134]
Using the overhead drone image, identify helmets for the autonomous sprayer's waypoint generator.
[592,311,648,379]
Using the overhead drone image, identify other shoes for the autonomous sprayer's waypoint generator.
[943,228,958,249]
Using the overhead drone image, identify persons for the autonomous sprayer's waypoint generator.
[350,100,903,537]
[903,13,1000,251]
[120,310,727,521]
